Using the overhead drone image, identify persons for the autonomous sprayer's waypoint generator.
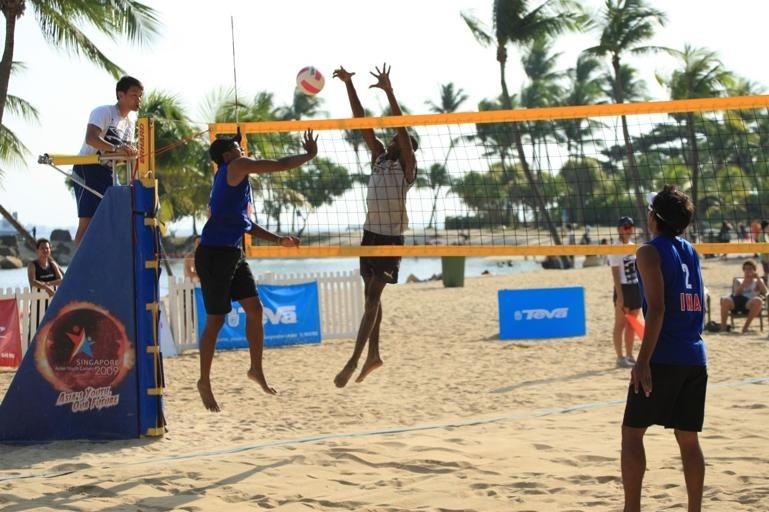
[183,234,201,280]
[734,220,744,245]
[717,258,769,333]
[605,216,645,369]
[620,186,709,512]
[564,223,578,269]
[758,220,769,288]
[73,76,145,255]
[580,225,592,245]
[716,219,733,260]
[329,61,422,389]
[26,238,64,344]
[192,126,320,413]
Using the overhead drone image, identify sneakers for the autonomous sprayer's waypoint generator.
[616,356,637,368]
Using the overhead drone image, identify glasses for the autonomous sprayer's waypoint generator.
[648,203,666,222]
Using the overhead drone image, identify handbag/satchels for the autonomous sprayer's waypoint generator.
[707,321,730,331]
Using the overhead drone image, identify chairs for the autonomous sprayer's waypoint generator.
[721,276,765,332]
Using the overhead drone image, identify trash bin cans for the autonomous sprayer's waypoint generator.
[441,257,466,287]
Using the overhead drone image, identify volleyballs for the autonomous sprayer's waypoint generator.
[296,67,324,96]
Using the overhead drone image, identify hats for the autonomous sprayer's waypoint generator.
[646,191,659,205]
[617,216,636,227]
[210,132,242,159]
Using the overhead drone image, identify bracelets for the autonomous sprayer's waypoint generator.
[111,145,121,153]
